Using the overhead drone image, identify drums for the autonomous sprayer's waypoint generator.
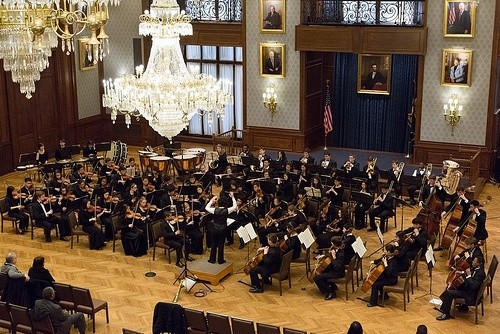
[105,140,128,167]
[138,148,207,179]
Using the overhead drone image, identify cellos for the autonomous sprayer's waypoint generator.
[361,248,401,294]
[307,241,347,284]
[415,169,485,302]
[396,228,421,248]
[242,223,304,276]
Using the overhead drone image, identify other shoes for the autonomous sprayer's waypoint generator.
[249,288,264,293]
[225,242,234,246]
[18,230,25,235]
[219,259,226,264]
[456,305,469,311]
[239,244,245,249]
[46,239,52,242]
[367,302,377,307]
[207,259,215,263]
[367,226,377,232]
[60,237,69,241]
[379,294,390,300]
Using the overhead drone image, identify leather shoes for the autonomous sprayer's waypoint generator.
[436,313,451,320]
[187,256,195,261]
[325,293,334,300]
[176,262,183,267]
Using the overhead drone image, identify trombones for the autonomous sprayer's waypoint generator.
[365,156,378,179]
[389,162,405,191]
[417,162,433,203]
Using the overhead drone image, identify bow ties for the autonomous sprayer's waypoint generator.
[430,185,434,187]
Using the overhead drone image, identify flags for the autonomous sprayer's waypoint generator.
[323,85,333,136]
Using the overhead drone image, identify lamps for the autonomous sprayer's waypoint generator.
[0,0,122,99]
[101,0,234,144]
[261,83,279,122]
[443,93,463,136]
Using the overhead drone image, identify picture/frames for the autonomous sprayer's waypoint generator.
[257,0,286,34]
[442,48,474,88]
[443,0,476,38]
[78,39,96,71]
[259,42,287,79]
[357,53,392,95]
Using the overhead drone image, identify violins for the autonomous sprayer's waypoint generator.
[13,171,298,230]
[298,198,306,213]
[319,203,329,221]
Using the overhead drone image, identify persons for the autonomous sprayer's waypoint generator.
[33,190,68,242]
[79,198,106,250]
[454,3,471,34]
[205,192,238,264]
[265,51,280,74]
[29,138,488,312]
[415,324,427,334]
[34,286,87,334]
[313,236,346,301]
[18,178,36,212]
[160,210,195,268]
[5,186,29,234]
[249,234,282,293]
[263,6,280,29]
[435,256,486,321]
[367,244,399,307]
[0,252,31,282]
[117,203,148,258]
[347,321,363,334]
[450,58,465,83]
[362,63,386,90]
[28,256,55,283]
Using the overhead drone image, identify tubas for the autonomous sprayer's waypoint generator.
[442,159,461,195]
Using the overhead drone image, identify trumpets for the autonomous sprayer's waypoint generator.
[345,159,355,174]
[322,156,330,169]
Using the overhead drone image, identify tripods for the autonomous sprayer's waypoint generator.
[173,151,214,293]
[296,229,313,281]
[236,226,253,273]
[414,244,440,308]
[245,223,258,258]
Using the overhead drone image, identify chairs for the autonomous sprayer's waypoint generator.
[0,144,499,334]
